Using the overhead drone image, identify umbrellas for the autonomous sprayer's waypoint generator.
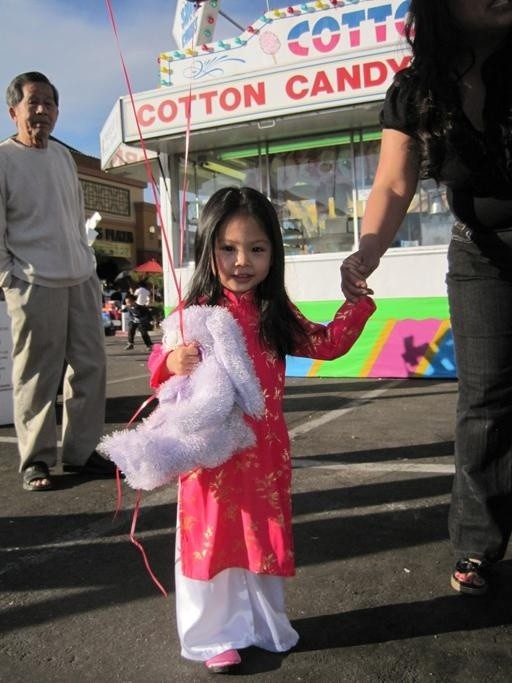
[132,257,162,300]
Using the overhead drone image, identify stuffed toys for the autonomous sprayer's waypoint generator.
[91,301,268,490]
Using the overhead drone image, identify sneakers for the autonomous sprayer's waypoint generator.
[205,649,242,672]
[450,556,493,595]
[146,347,152,351]
[123,345,134,350]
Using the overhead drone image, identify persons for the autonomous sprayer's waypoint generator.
[143,184,376,676]
[117,291,155,350]
[129,282,152,306]
[338,0,510,596]
[0,72,128,491]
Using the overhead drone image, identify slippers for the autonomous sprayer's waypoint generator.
[63,450,116,473]
[23,461,53,491]
